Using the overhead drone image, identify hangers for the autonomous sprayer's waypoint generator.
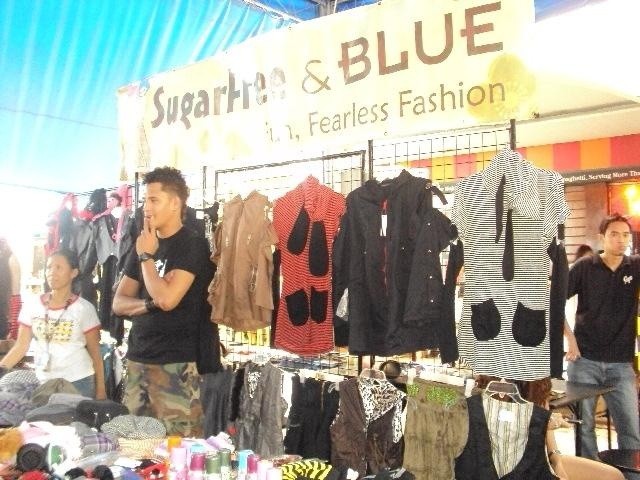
[481,378,528,406]
[403,356,478,398]
[249,353,269,368]
[375,168,449,206]
[327,368,408,401]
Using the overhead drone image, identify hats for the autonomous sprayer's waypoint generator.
[0,369,167,439]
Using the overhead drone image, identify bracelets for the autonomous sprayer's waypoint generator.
[144,296,158,314]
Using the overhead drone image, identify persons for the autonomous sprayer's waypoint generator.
[557,212,640,457]
[110,164,212,436]
[1,236,19,343]
[0,248,109,401]
[369,359,409,384]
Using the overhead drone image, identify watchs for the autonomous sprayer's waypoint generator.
[138,251,154,263]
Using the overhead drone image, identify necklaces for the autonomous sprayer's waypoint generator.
[44,291,74,344]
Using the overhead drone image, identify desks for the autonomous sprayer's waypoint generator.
[547,376,616,461]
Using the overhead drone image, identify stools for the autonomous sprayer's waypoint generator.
[597,449,640,475]
